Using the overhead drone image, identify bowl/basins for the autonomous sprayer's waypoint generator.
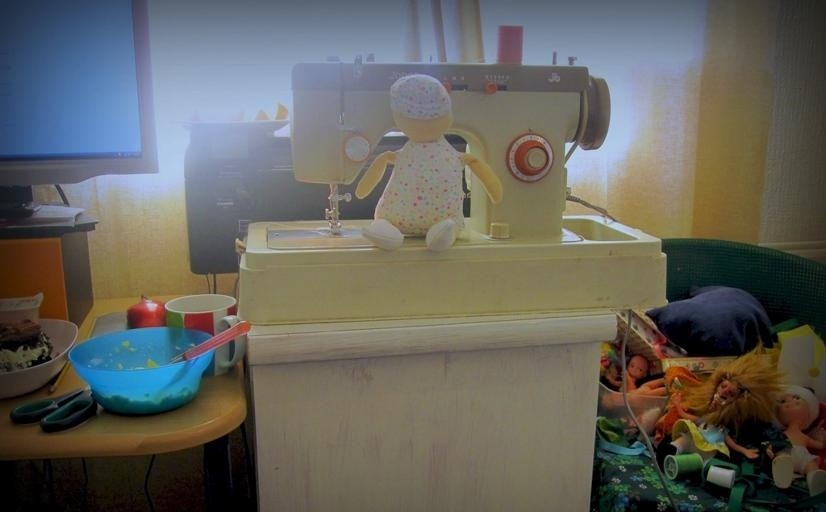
[69,325,215,417]
[1,320,79,401]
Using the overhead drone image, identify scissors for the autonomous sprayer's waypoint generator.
[9,384,98,433]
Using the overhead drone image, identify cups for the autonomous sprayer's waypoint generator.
[165,294,245,375]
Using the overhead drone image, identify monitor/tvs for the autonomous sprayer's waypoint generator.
[1,1,161,225]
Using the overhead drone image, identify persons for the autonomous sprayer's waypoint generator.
[598,356,825,496]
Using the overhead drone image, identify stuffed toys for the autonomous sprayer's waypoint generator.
[353,73,502,252]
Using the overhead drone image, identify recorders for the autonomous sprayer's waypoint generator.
[184,119,470,275]
